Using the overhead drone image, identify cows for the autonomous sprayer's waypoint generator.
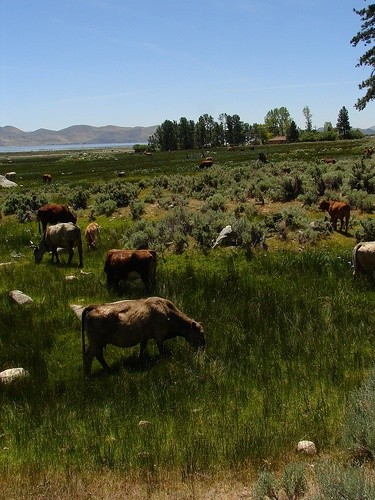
[41,173,52,183]
[81,297,206,377]
[321,158,336,164]
[85,222,102,252]
[318,196,351,233]
[199,161,213,169]
[33,222,84,268]
[352,242,375,289]
[104,249,156,295]
[37,203,77,234]
